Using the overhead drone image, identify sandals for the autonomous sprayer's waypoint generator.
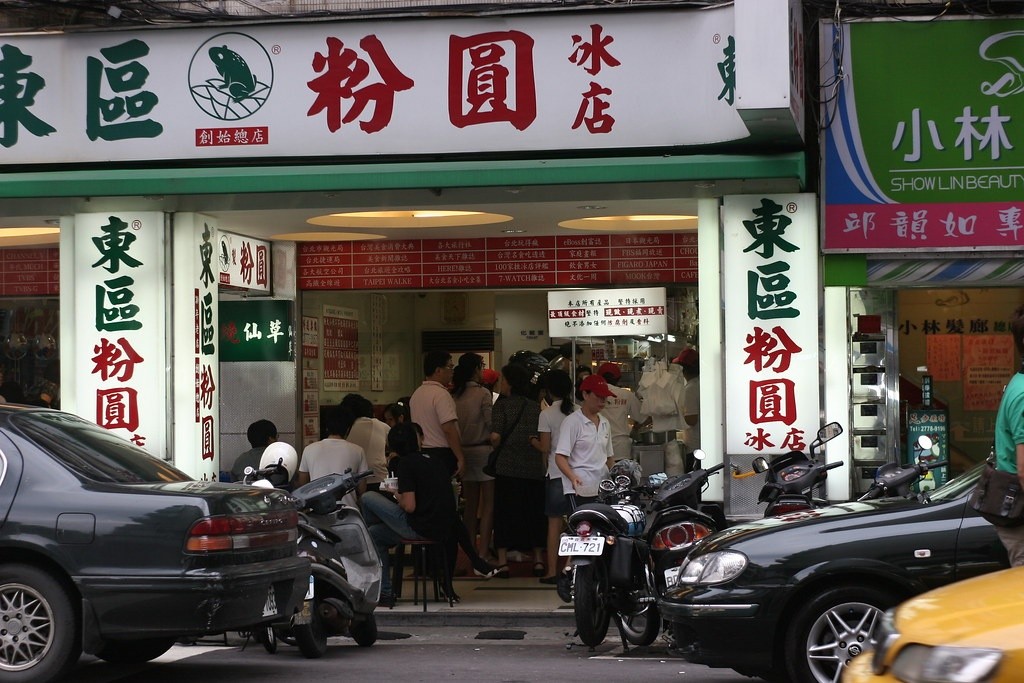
[498,564,509,579]
[533,561,544,576]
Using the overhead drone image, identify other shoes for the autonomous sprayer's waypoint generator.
[556,569,572,602]
[539,575,556,583]
[377,596,396,607]
[473,566,504,578]
[439,585,459,602]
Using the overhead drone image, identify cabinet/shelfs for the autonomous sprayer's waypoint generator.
[824,285,902,505]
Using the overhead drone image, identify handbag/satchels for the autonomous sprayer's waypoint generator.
[972,460,1024,528]
[482,448,500,478]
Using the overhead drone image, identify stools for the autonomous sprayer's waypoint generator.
[389,540,453,612]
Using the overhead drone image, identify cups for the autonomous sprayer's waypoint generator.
[384,478,398,489]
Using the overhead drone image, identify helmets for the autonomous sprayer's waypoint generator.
[259,440,298,483]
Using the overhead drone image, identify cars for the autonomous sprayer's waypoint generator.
[659,460,1012,683]
[0,401,312,683]
[837,561,1024,683]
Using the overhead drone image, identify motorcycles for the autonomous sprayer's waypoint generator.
[557,461,660,658]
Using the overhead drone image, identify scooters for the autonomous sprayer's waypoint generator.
[639,446,725,593]
[226,459,384,659]
[854,434,948,498]
[751,421,844,519]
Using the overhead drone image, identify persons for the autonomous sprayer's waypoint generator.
[34,382,60,410]
[231,345,698,608]
[989,308,1024,568]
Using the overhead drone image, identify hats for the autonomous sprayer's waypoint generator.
[598,362,621,378]
[672,348,699,363]
[579,374,619,398]
